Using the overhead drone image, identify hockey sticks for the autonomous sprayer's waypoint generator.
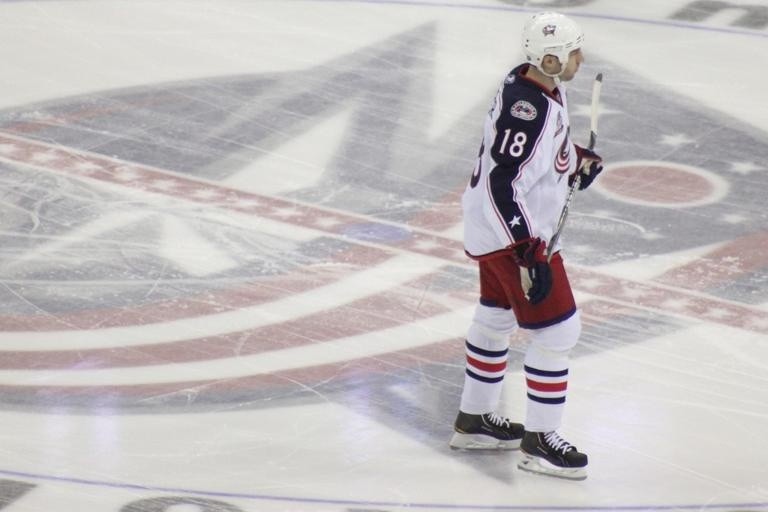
[546,74,601,264]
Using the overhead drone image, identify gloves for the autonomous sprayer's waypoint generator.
[567,144,604,191]
[508,236,552,306]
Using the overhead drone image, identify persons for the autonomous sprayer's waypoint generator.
[453,10,606,471]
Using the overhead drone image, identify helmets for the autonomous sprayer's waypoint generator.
[521,10,585,77]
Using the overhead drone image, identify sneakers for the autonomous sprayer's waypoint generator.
[519,430,588,468]
[453,410,525,441]
